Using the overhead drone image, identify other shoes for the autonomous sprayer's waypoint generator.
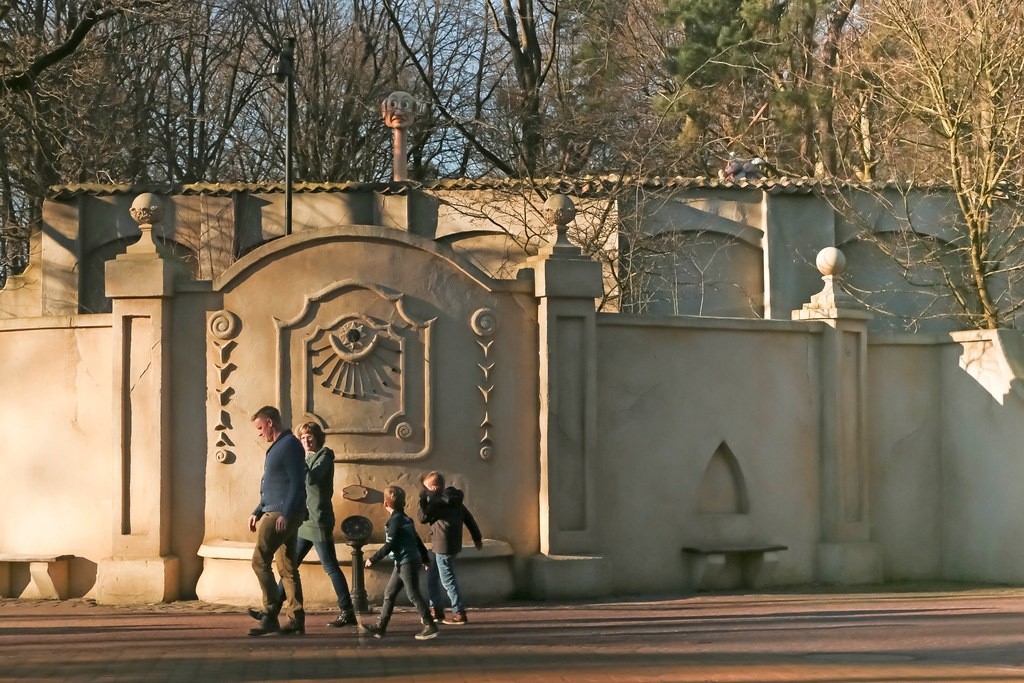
[414,624,440,640]
[326,615,358,627]
[247,617,279,635]
[419,615,442,623]
[358,623,385,638]
[442,614,467,625]
[247,608,262,620]
[278,622,305,635]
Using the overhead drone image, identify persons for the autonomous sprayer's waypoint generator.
[247,421,357,627]
[416,471,484,625]
[359,486,440,640]
[246,406,311,637]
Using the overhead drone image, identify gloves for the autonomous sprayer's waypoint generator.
[419,490,427,500]
[474,541,483,551]
